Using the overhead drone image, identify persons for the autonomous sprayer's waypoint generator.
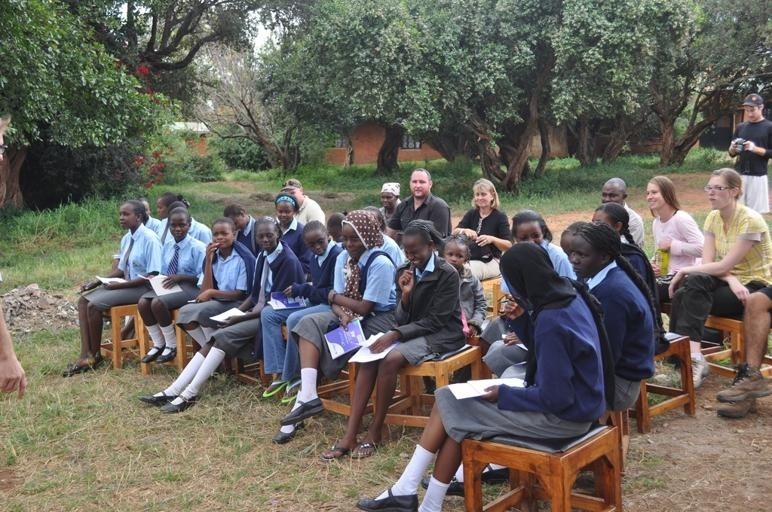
[728,94,772,214]
[63,169,510,461]
[476,203,658,486]
[603,168,772,419]
[357,244,605,512]
[0,315,26,400]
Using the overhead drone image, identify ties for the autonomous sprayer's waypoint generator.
[166,245,180,276]
[122,238,134,280]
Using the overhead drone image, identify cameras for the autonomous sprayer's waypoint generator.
[735,138,745,153]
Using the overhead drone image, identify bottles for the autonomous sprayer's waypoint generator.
[653,246,670,279]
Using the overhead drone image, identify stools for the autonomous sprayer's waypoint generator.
[100,276,771,512]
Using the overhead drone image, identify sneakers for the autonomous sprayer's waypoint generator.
[716,361,772,418]
[653,353,711,390]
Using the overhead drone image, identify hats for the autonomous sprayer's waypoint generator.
[742,94,764,107]
[280,178,302,192]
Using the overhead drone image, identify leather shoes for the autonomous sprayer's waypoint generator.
[141,343,176,364]
[137,391,196,414]
[421,468,509,497]
[356,488,418,512]
[61,350,106,377]
[272,397,325,444]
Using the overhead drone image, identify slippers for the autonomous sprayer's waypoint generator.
[262,379,301,404]
[319,441,382,462]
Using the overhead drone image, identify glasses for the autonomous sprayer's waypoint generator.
[703,185,733,192]
[282,181,301,188]
